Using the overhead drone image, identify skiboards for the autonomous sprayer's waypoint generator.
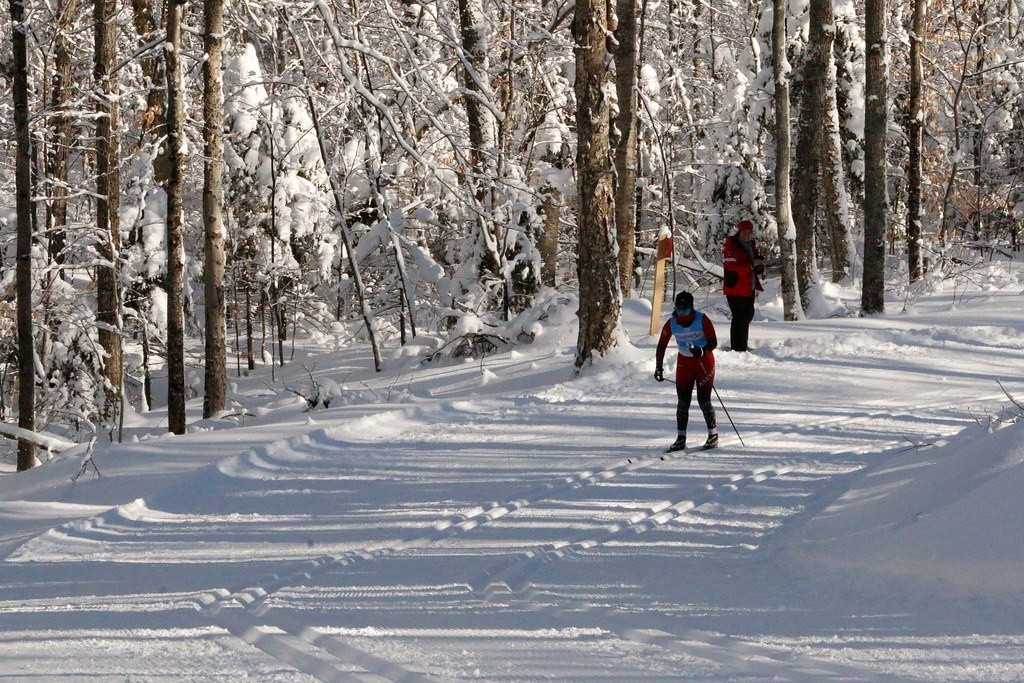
[628,446,718,464]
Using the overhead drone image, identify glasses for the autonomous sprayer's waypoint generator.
[676,308,691,315]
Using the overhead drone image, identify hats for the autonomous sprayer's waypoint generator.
[675,290,694,308]
[738,221,753,234]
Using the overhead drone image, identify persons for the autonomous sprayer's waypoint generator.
[654,290,719,450]
[722,220,767,352]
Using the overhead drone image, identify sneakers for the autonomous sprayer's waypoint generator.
[668,435,686,452]
[701,433,718,451]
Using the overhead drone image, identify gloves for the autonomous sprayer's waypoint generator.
[754,265,766,280]
[690,346,702,359]
[724,270,739,281]
[654,369,664,382]
